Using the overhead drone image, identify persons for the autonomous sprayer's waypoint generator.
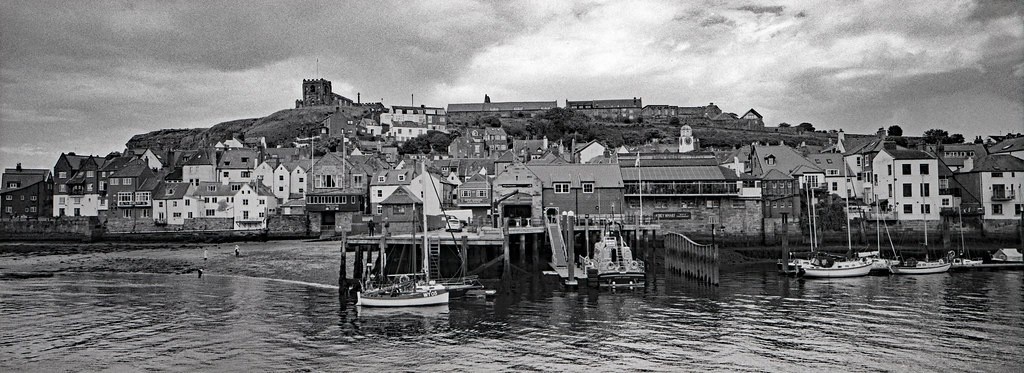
[234,244,241,258]
[959,249,964,265]
[8,212,20,222]
[203,248,208,263]
[367,218,375,236]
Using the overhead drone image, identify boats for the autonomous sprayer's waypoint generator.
[589,202,646,288]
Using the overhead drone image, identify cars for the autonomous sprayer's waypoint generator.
[600,218,608,225]
[445,218,467,233]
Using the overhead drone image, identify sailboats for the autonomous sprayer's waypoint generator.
[356,201,450,308]
[775,181,1024,279]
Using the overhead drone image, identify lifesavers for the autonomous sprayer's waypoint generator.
[630,262,639,269]
[606,261,614,270]
